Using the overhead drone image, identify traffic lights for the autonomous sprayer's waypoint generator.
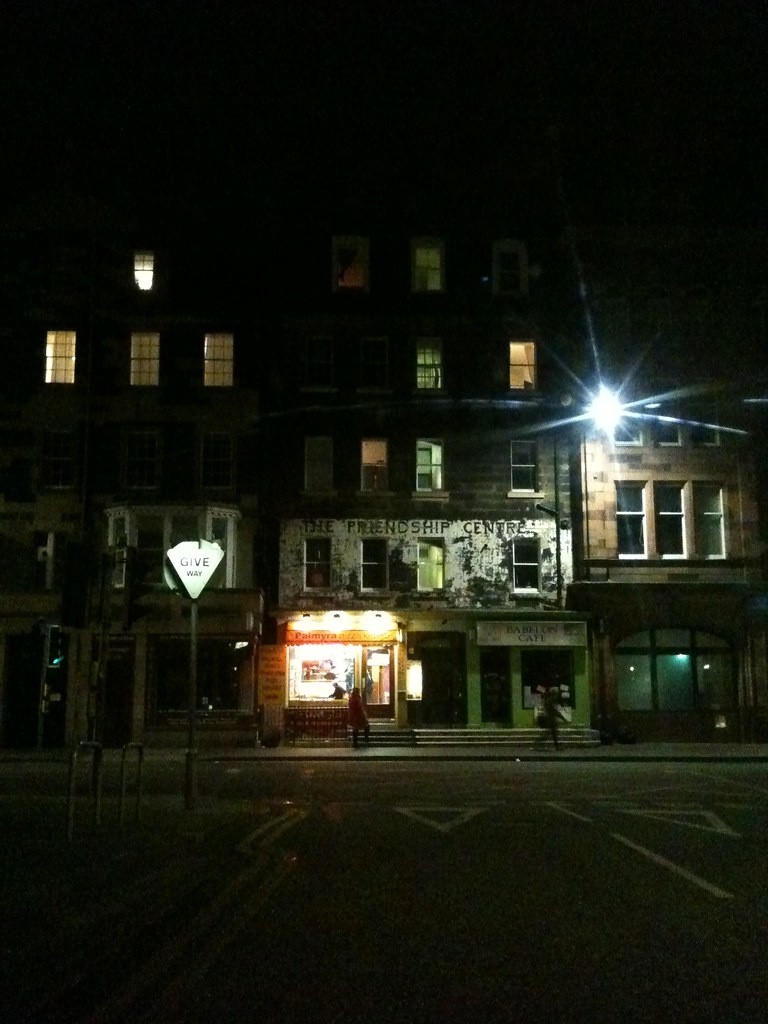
[48,627,65,665]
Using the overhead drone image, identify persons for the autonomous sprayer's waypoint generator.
[534,687,562,752]
[327,683,347,700]
[348,688,375,748]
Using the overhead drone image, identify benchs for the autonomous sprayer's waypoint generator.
[157,705,263,750]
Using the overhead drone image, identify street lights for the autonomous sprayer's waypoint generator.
[555,390,625,611]
